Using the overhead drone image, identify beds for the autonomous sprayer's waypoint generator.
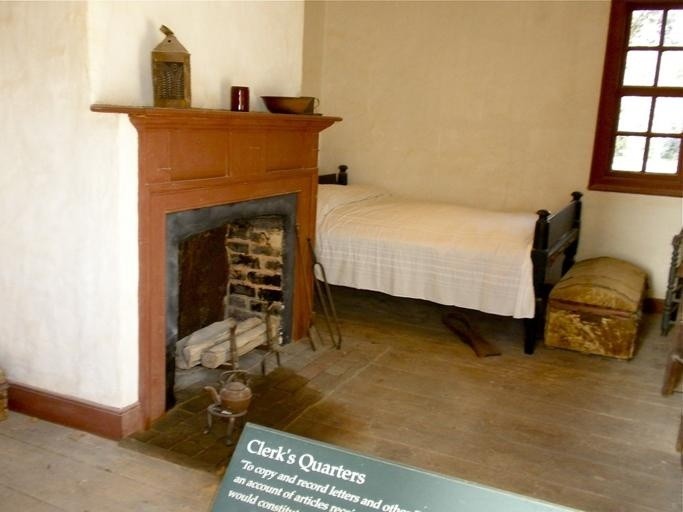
[318,164,582,355]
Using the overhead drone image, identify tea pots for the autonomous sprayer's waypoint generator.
[203,370,252,414]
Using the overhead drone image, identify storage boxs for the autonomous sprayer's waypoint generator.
[545,258,649,357]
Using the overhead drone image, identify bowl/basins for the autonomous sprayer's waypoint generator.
[260,96,312,114]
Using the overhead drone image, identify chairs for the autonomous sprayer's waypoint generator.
[660,228,683,337]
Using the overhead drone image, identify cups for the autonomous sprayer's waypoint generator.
[300,96,320,114]
[230,86,250,112]
